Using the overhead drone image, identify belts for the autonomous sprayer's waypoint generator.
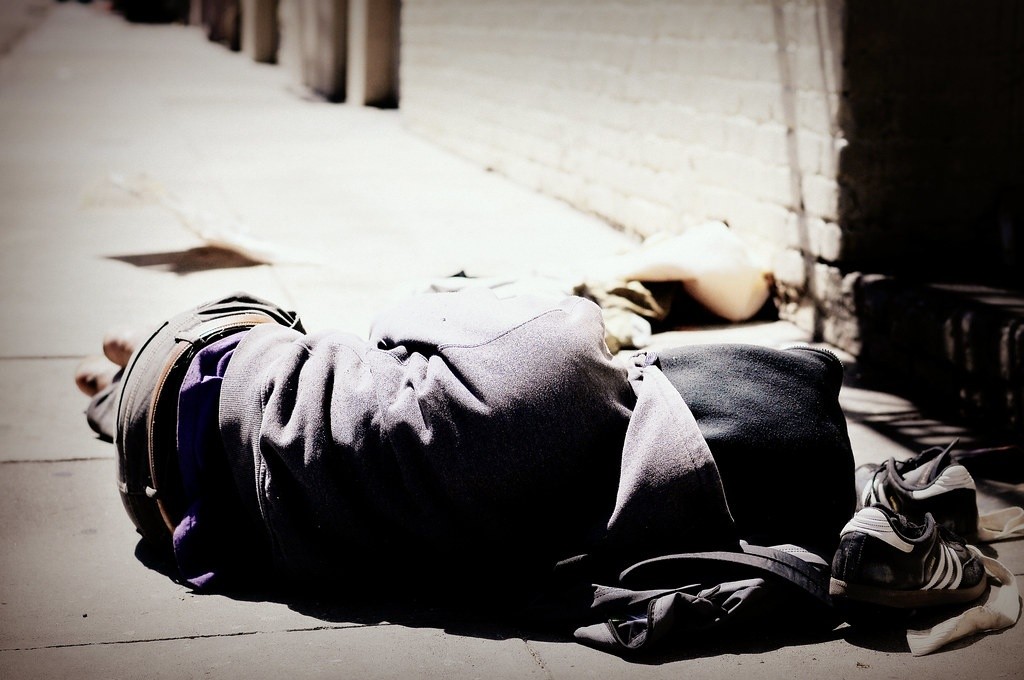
[145,312,273,538]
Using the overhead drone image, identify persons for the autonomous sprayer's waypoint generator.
[74,291,855,624]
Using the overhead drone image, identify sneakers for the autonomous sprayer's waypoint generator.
[853,444,977,540]
[831,502,986,606]
